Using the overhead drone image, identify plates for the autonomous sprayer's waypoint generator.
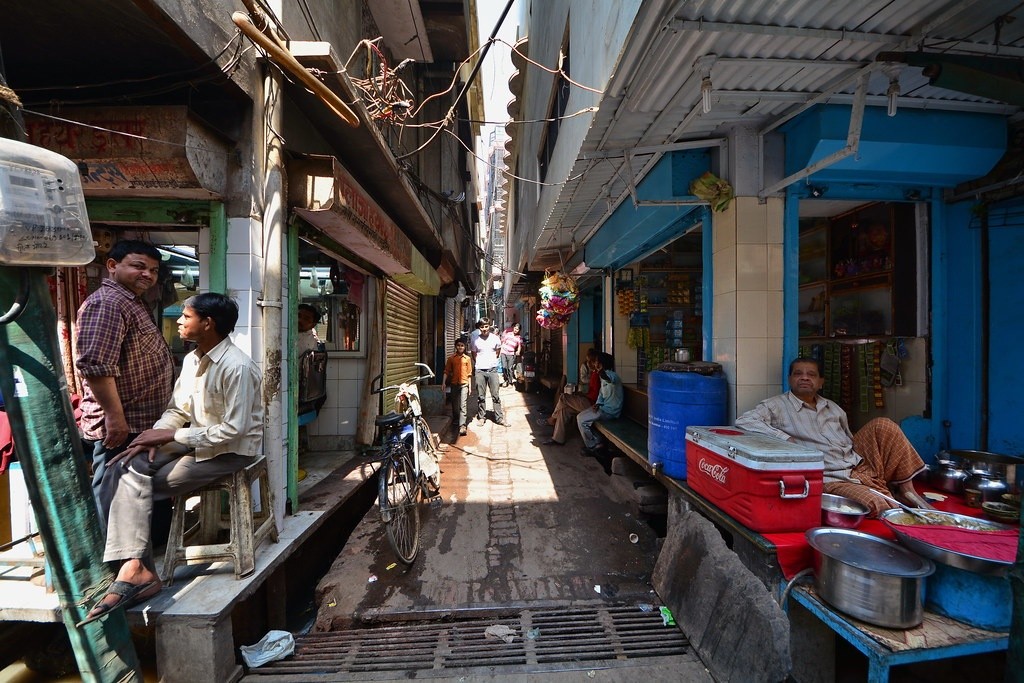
[1002,493,1020,505]
[982,501,1019,519]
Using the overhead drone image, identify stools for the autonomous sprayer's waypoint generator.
[158,452,279,589]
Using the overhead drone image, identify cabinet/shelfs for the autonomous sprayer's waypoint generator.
[798,204,917,339]
[638,268,703,391]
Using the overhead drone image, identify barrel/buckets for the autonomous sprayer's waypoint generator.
[647,361,728,479]
[926,563,1012,630]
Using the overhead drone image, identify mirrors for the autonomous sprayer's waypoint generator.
[298,265,368,359]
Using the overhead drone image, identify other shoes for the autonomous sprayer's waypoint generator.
[452,423,468,435]
[496,419,512,427]
[581,444,607,457]
[502,381,513,389]
[477,418,486,427]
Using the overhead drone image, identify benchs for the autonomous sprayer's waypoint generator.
[592,415,836,683]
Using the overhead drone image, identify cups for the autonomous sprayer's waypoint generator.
[965,489,983,508]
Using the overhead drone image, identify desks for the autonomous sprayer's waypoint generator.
[779,576,1010,683]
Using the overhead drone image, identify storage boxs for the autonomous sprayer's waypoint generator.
[685,426,824,531]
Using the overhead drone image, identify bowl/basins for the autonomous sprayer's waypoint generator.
[880,507,1014,573]
[822,493,871,528]
[809,543,922,628]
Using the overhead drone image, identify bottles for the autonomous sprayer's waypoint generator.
[566,383,573,394]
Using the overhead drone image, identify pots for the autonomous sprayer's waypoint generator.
[931,450,1024,501]
[675,348,692,363]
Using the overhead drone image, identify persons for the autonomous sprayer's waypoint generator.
[576,352,623,456]
[441,339,472,435]
[297,303,321,361]
[472,317,511,427]
[470,322,523,387]
[85,292,263,618]
[72,238,176,573]
[734,357,938,519]
[536,348,600,445]
[578,360,593,393]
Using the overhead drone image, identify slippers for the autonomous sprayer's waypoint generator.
[537,418,555,427]
[543,438,566,445]
[72,581,161,628]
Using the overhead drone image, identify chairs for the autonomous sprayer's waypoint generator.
[296,350,328,426]
